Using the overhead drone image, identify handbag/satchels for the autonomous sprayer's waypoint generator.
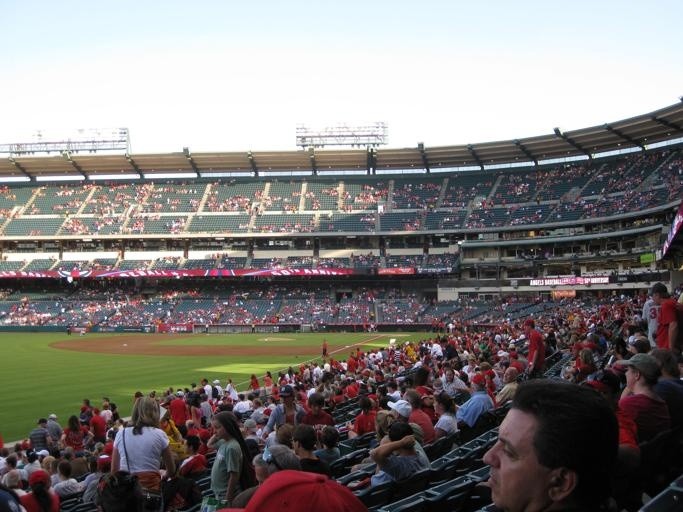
[139,488,163,512]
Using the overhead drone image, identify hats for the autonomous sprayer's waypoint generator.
[615,354,661,382]
[36,450,49,457]
[278,385,292,397]
[36,418,47,423]
[176,380,220,397]
[218,469,372,512]
[523,319,534,326]
[471,376,486,386]
[29,470,49,487]
[647,281,667,296]
[388,400,412,418]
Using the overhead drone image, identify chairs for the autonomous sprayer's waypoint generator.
[0,142,441,331]
[63,373,681,512]
[441,148,680,372]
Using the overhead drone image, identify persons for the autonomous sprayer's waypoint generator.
[0,143,682,512]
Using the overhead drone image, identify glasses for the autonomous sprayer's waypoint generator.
[263,445,282,471]
